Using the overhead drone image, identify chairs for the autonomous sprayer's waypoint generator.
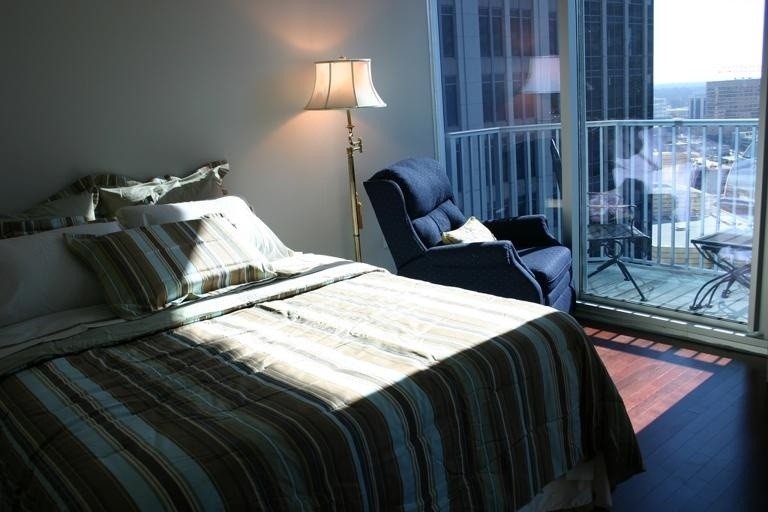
[363,153,578,319]
[550,139,654,305]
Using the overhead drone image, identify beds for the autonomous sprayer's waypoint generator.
[0,153,622,511]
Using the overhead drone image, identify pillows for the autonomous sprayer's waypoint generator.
[64,212,278,323]
[0,217,123,323]
[115,194,295,266]
[4,192,101,224]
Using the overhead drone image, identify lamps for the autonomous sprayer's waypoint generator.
[302,58,390,264]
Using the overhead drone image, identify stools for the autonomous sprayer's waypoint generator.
[688,231,753,310]
